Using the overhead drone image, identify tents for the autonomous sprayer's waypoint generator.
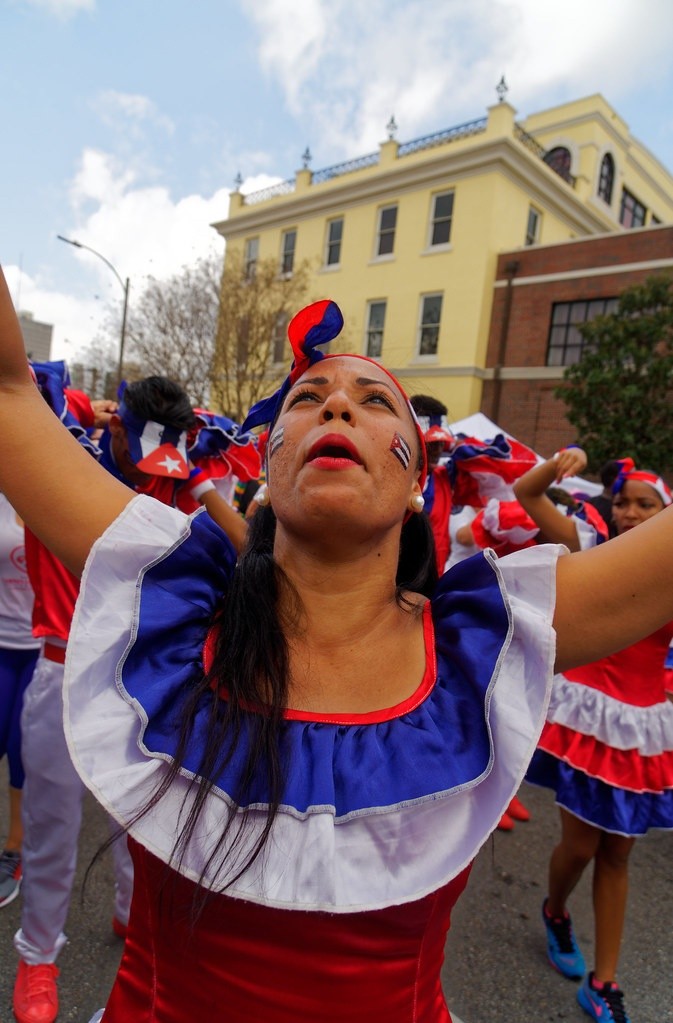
[445,410,604,503]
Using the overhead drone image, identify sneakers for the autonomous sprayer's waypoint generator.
[542,897,586,980]
[576,971,631,1023]
[13,957,60,1023]
[0,849,24,908]
[112,916,128,941]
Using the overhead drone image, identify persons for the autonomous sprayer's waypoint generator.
[0,265,673,1023]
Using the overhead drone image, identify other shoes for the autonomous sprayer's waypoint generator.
[506,795,530,821]
[497,813,514,832]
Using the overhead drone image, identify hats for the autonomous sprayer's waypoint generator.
[415,415,456,443]
[117,380,190,480]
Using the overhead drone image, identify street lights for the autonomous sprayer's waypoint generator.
[56,235,130,400]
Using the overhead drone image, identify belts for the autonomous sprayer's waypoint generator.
[44,642,66,664]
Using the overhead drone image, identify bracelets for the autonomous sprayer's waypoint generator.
[186,468,216,499]
[553,444,579,460]
